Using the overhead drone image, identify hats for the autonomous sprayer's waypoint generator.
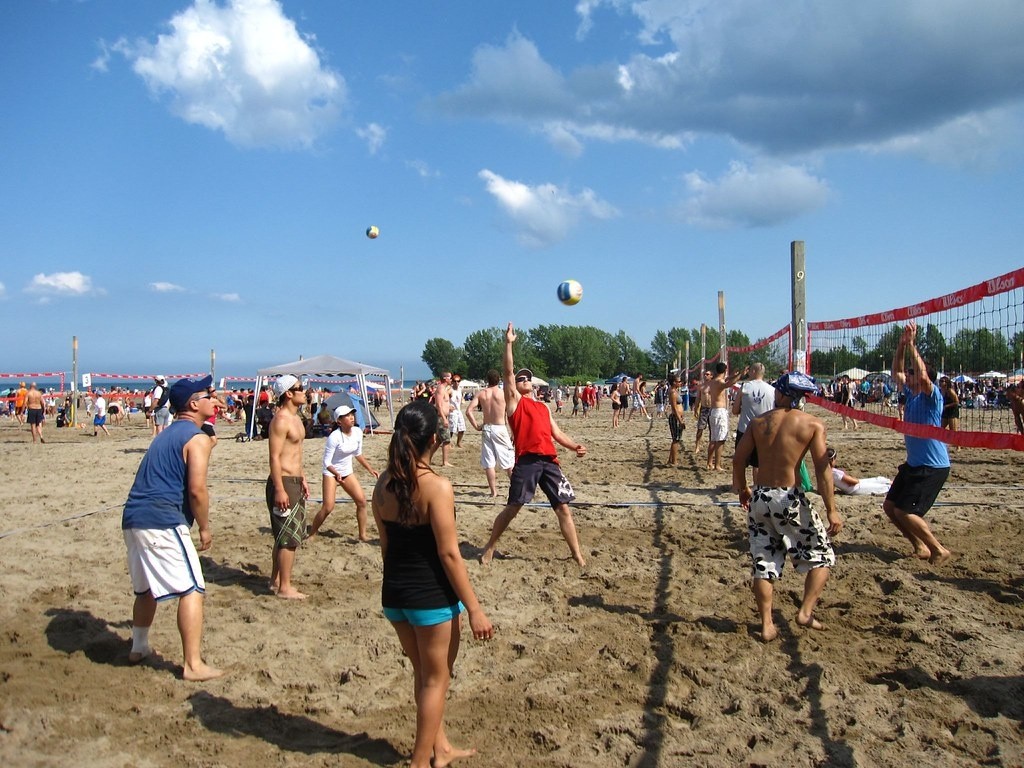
[154,375,166,383]
[515,368,533,378]
[452,375,460,379]
[170,375,212,410]
[772,371,818,408]
[273,375,299,398]
[334,406,357,422]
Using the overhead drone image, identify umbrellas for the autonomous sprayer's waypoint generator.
[529,377,549,387]
[949,374,979,399]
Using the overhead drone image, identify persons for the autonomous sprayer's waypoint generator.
[266,376,310,599]
[306,406,379,543]
[705,362,750,471]
[883,319,951,565]
[479,322,588,568]
[540,373,668,428]
[410,373,466,468]
[664,374,688,467]
[692,370,714,455]
[122,375,224,681]
[0,374,388,450]
[733,371,843,641]
[768,370,1024,453]
[372,401,493,768]
[465,370,517,498]
[827,448,893,496]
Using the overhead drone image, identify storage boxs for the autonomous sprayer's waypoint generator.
[131,408,138,413]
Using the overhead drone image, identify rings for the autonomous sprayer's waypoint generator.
[479,637,484,640]
[485,635,489,639]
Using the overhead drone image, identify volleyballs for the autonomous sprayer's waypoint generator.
[557,280,583,306]
[366,225,379,238]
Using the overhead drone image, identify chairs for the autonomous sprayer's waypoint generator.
[317,413,329,436]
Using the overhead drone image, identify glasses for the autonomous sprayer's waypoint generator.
[907,369,916,374]
[516,377,531,382]
[192,392,211,402]
[289,385,304,392]
[453,380,461,382]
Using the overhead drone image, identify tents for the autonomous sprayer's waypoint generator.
[605,372,638,395]
[249,354,395,440]
[836,368,872,379]
[452,379,480,404]
[979,370,1007,389]
[935,371,949,386]
[854,373,894,403]
